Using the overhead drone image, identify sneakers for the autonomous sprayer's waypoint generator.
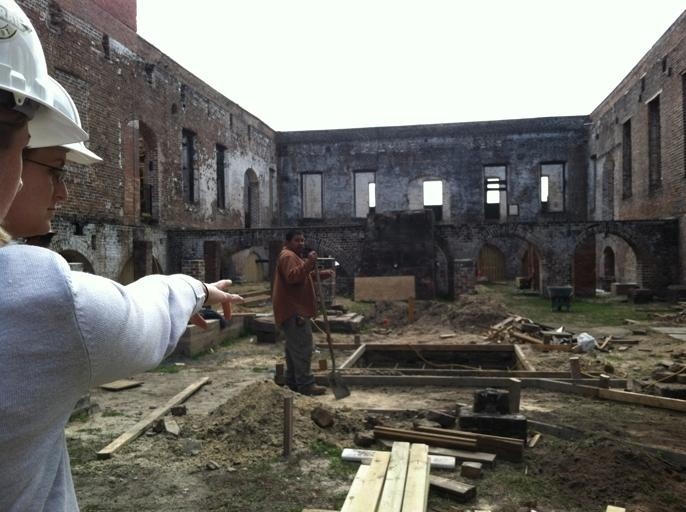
[305,384,326,395]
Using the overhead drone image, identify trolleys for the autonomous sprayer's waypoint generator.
[547,285,574,311]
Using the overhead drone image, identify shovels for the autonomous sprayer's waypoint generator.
[314,263,350,400]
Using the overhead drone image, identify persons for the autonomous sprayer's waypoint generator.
[0,0,247,512]
[271,231,335,397]
[0,72,107,246]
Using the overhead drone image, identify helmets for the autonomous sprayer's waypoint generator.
[0,0,103,165]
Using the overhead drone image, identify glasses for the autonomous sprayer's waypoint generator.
[22,158,67,183]
